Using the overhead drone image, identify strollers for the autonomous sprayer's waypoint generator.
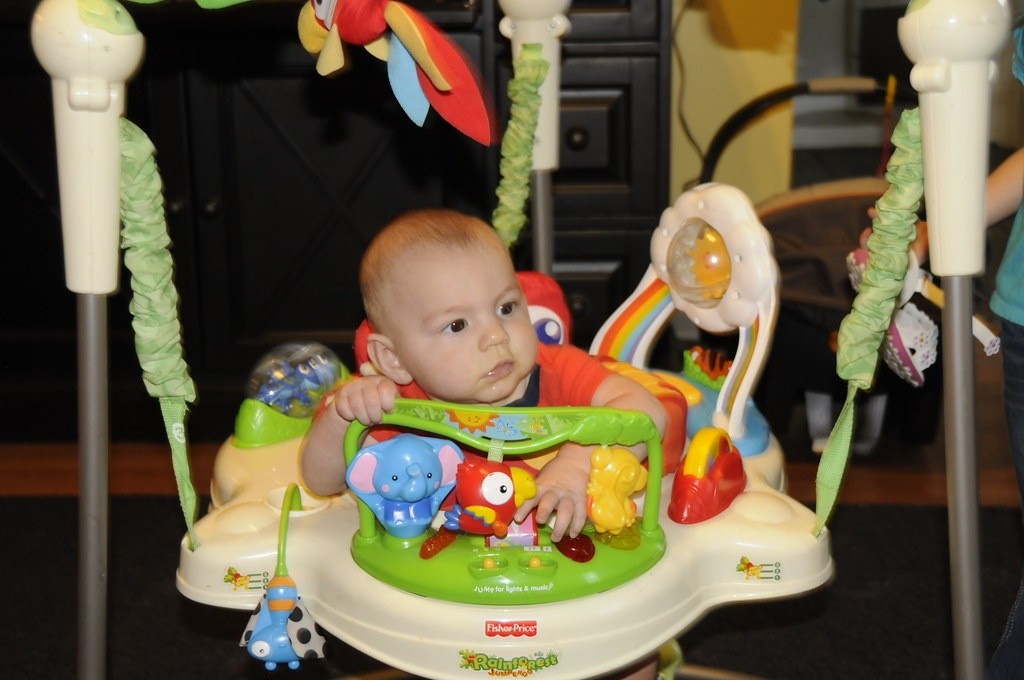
[603,73,944,466]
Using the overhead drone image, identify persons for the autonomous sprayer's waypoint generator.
[860,10,1024,504]
[301,212,668,680]
